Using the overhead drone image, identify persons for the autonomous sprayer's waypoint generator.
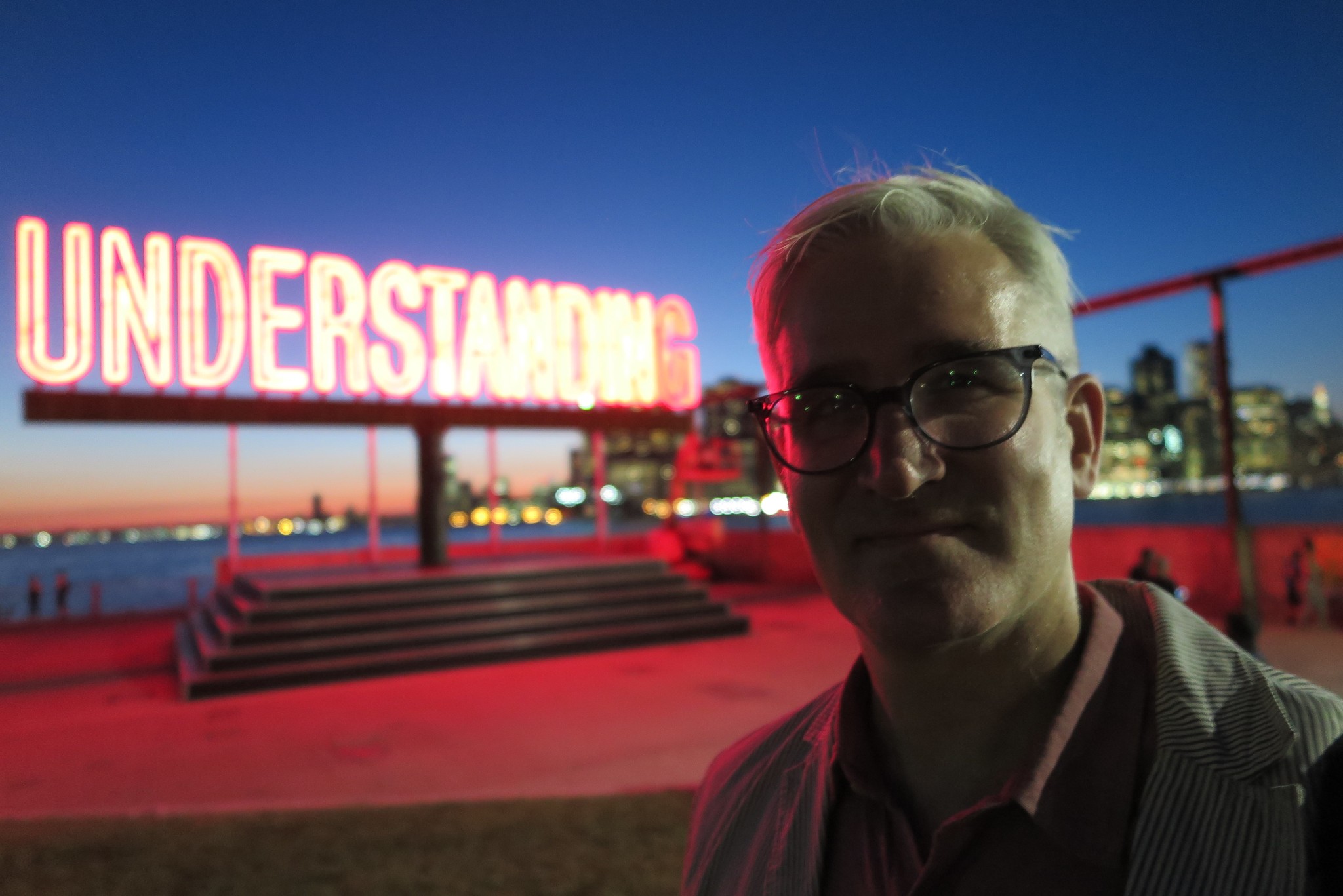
[1127,547,1322,627]
[684,165,1343,896]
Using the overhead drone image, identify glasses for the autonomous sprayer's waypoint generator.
[744,342,1070,476]
